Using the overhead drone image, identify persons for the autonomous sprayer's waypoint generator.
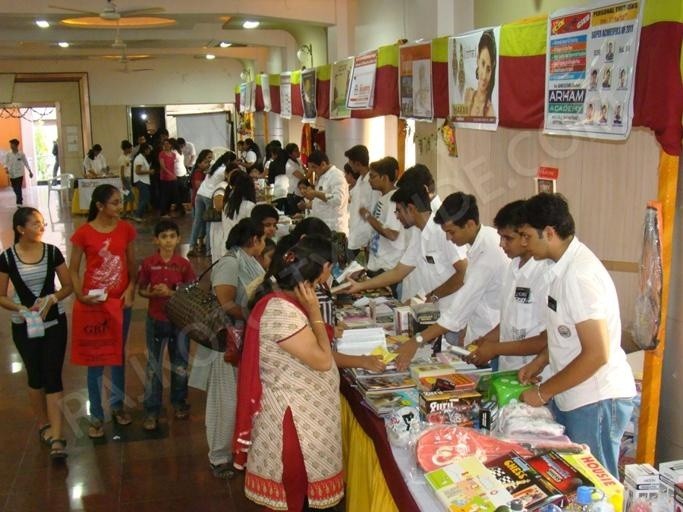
[3,139,33,207]
[53,139,58,178]
[0,208,74,459]
[83,143,110,179]
[586,43,627,124]
[69,185,141,439]
[464,29,497,119]
[119,128,636,511]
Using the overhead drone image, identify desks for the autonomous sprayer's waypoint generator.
[253,178,626,512]
[71,176,192,217]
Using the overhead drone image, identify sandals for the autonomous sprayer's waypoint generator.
[39,425,67,446]
[210,462,235,478]
[50,437,68,460]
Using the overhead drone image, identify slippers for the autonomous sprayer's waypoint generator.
[112,410,132,425]
[88,423,103,438]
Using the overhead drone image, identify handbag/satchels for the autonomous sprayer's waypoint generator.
[164,283,234,352]
[203,209,222,222]
[70,299,124,366]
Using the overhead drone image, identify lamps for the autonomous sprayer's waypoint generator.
[35,19,49,29]
[242,20,260,29]
[297,44,313,67]
[220,42,232,48]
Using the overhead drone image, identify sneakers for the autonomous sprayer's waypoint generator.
[143,416,157,430]
[176,409,190,418]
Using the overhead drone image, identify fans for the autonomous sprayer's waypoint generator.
[48,0,165,73]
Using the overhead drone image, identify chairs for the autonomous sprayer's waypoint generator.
[212,146,238,162]
[48,173,75,208]
